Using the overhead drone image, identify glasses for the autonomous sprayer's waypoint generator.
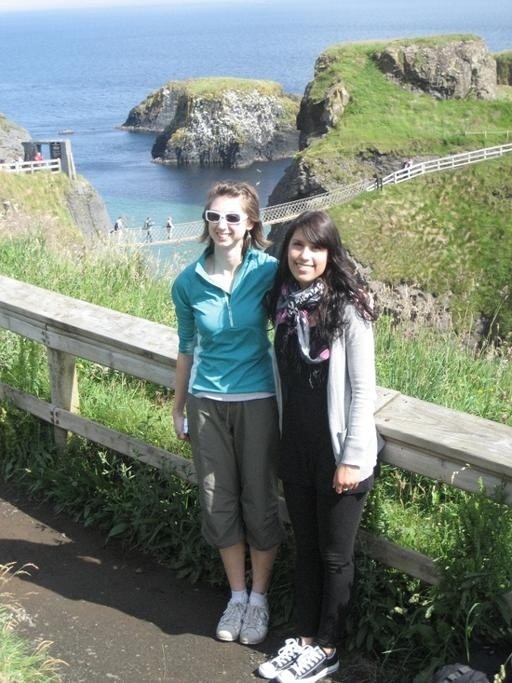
[204,210,247,224]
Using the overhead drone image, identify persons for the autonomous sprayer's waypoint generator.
[34,152,44,172]
[142,216,154,243]
[165,218,175,240]
[256,210,379,683]
[114,216,124,230]
[170,180,283,647]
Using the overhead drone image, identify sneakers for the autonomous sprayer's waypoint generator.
[258,637,306,679]
[240,600,270,645]
[217,600,248,641]
[278,645,340,683]
